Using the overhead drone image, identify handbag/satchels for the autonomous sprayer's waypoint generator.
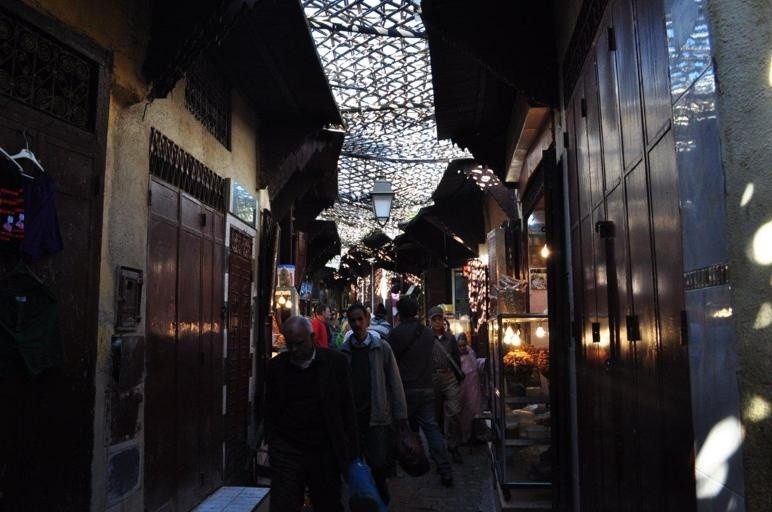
[398,425,431,478]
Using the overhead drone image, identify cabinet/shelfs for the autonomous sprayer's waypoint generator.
[487,314,550,501]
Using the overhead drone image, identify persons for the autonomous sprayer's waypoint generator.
[285,294,489,489]
[258,314,357,508]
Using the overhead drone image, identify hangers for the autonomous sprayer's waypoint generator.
[6,127,44,179]
[0,147,24,173]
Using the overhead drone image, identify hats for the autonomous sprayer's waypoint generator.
[428,306,443,320]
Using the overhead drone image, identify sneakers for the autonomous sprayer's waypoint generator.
[440,473,453,487]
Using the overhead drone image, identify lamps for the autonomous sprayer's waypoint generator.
[366,172,396,227]
